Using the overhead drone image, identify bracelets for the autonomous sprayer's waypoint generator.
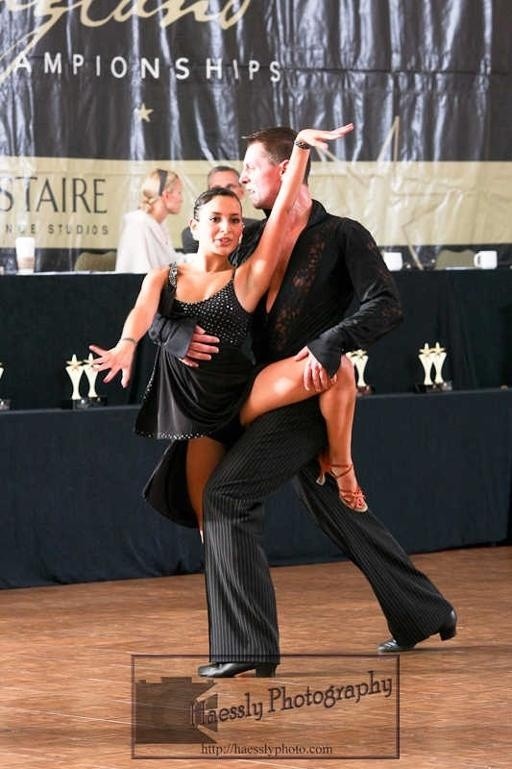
[294,141,310,149]
[120,338,136,345]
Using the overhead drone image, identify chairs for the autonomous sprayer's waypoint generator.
[73,249,121,272]
[434,249,477,269]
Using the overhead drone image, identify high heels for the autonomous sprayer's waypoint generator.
[378,609,456,652]
[317,451,368,512]
[199,662,275,678]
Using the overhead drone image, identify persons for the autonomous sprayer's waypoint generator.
[114,169,185,274]
[198,127,458,677]
[181,166,261,252]
[88,123,369,546]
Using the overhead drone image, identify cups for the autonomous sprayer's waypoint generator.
[14,237,37,274]
[382,252,403,272]
[473,250,497,270]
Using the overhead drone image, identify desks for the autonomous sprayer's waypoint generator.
[2,269,512,405]
[1,380,512,594]
